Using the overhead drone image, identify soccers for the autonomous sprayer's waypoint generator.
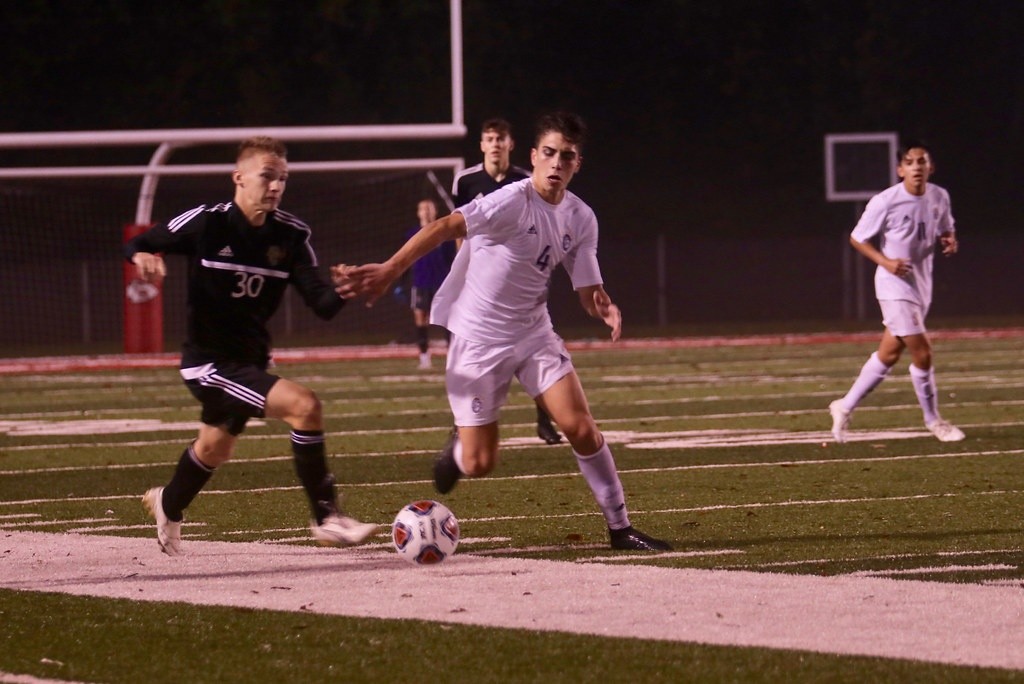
[392,498,461,567]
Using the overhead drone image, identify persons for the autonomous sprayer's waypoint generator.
[122,137,378,556]
[829,139,966,442]
[404,118,562,448]
[335,112,673,552]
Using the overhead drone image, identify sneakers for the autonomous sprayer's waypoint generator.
[311,514,379,546]
[435,425,462,494]
[927,420,966,442]
[143,487,185,557]
[829,399,851,444]
[609,526,674,553]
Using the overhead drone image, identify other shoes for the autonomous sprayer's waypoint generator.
[536,424,563,445]
[418,352,432,370]
